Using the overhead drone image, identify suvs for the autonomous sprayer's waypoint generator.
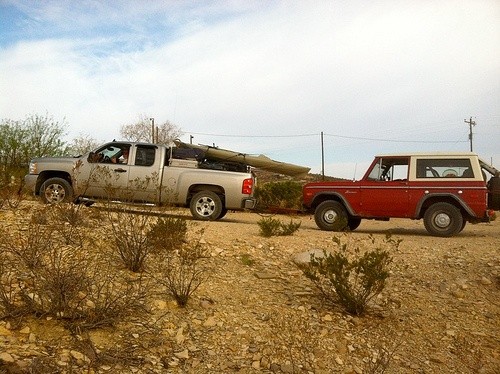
[301,144,500,235]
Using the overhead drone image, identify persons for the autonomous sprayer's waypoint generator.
[112,147,129,164]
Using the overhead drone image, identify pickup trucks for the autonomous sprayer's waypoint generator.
[25,137,258,219]
